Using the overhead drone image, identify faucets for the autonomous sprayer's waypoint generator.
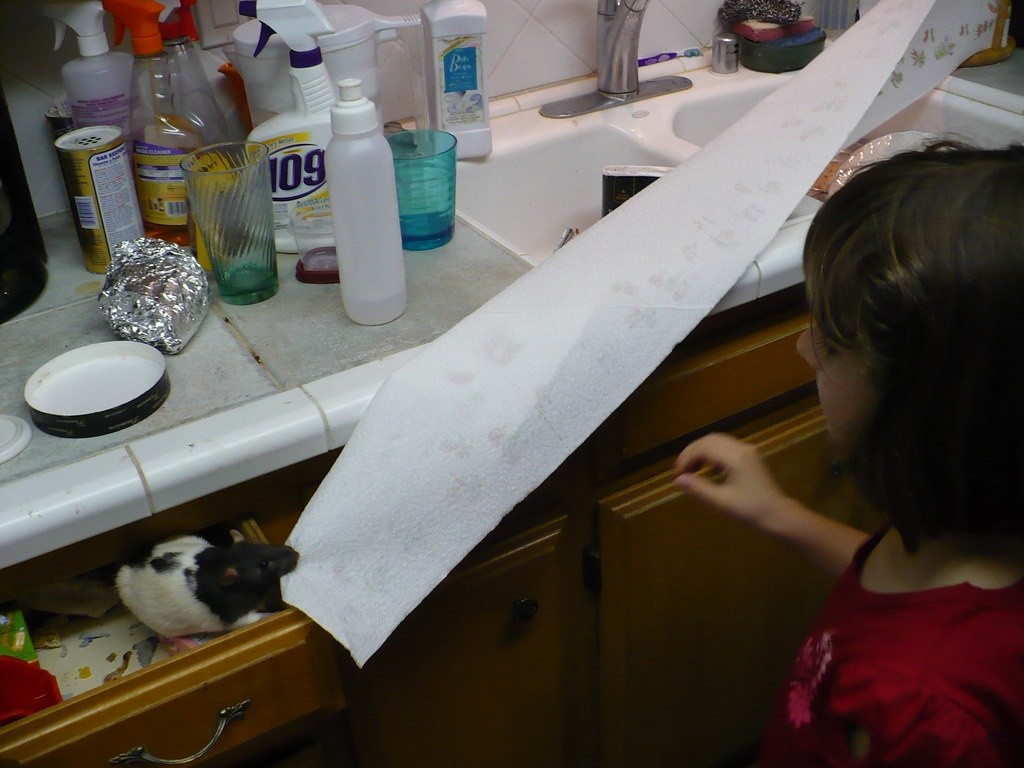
[594,1,647,99]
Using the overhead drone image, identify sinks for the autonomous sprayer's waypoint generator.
[455,123,826,330]
[671,72,1021,219]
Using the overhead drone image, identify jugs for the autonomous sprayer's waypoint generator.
[215,62,263,140]
[220,5,432,165]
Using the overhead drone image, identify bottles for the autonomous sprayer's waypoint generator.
[324,77,405,325]
[418,0,492,160]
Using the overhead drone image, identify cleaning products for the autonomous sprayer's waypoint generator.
[37,0,338,260]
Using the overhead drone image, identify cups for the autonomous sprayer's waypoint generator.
[385,129,457,251]
[289,200,344,272]
[179,141,279,305]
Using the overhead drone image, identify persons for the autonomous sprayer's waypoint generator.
[669,139,1024,768]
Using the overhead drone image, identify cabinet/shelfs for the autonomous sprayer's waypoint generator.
[1,275,883,768]
